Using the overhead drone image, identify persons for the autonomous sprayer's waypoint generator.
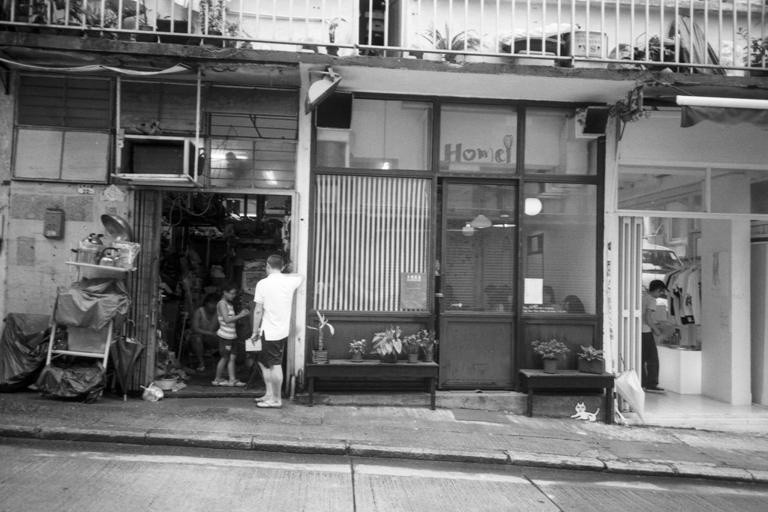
[190,292,220,371]
[251,254,305,408]
[445,284,586,313]
[212,281,249,386]
[642,280,666,393]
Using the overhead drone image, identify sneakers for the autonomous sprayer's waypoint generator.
[647,386,665,393]
[212,377,247,386]
[255,397,281,408]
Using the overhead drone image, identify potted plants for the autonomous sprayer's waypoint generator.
[307,309,334,366]
[200,2,238,48]
[347,327,438,362]
[419,25,487,66]
[736,27,768,88]
[576,344,605,373]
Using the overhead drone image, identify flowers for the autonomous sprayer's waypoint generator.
[532,339,569,359]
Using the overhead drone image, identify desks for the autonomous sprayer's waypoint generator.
[520,367,615,419]
[306,360,438,408]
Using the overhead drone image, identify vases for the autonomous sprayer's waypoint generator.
[542,356,557,373]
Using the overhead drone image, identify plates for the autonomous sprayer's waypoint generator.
[101,213,133,242]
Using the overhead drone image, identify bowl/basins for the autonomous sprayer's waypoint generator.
[154,375,179,391]
[98,247,120,266]
